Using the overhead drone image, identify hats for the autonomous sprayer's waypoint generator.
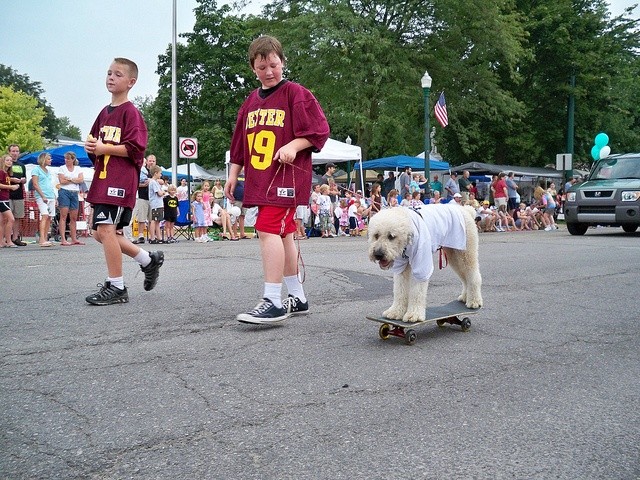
[326,163,337,167]
[451,172,458,175]
[483,200,489,205]
[454,193,463,197]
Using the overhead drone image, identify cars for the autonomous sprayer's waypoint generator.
[564,153,640,235]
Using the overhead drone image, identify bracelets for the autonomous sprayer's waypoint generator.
[70,178,72,182]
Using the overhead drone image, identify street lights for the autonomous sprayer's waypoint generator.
[421,71,432,195]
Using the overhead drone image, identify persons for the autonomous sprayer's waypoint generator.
[0,155,19,249]
[175,178,191,222]
[200,180,214,243]
[494,172,509,203]
[371,183,381,213]
[506,172,519,205]
[489,174,498,204]
[140,158,148,184]
[296,185,369,237]
[401,191,413,205]
[224,36,331,326]
[447,193,462,204]
[534,186,557,231]
[321,163,337,184]
[547,183,557,222]
[136,154,156,244]
[413,192,423,206]
[564,179,576,193]
[8,144,26,247]
[413,174,428,193]
[228,167,252,240]
[383,171,403,199]
[163,175,171,190]
[148,165,165,244]
[464,193,480,208]
[29,178,42,221]
[32,153,58,247]
[74,158,80,165]
[84,57,164,306]
[191,190,208,243]
[458,169,474,200]
[163,186,180,243]
[375,173,384,189]
[211,178,224,208]
[387,188,399,208]
[419,175,427,187]
[445,171,461,202]
[473,199,545,232]
[208,192,239,241]
[428,175,444,196]
[400,166,412,199]
[57,151,85,246]
[430,190,445,204]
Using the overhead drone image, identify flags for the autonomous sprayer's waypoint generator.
[433,94,448,128]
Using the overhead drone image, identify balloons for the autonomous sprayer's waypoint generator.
[591,133,611,160]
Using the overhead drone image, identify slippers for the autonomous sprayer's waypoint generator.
[5,243,19,248]
[0,243,5,248]
[60,242,71,245]
[321,235,328,238]
[71,241,85,245]
[239,235,251,239]
[234,235,239,239]
[328,235,334,238]
[39,241,54,246]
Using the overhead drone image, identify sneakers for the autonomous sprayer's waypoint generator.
[494,228,501,232]
[512,228,520,231]
[136,237,145,243]
[150,239,161,244]
[505,228,513,231]
[140,250,164,291]
[223,232,227,236]
[544,226,552,231]
[231,236,237,240]
[158,240,168,244]
[551,225,557,231]
[85,281,129,305]
[167,238,176,243]
[195,237,214,242]
[237,298,287,324]
[499,228,506,232]
[12,239,27,246]
[283,295,309,314]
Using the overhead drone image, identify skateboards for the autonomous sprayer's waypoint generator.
[365,298,483,344]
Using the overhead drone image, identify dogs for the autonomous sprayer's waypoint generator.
[366,204,483,324]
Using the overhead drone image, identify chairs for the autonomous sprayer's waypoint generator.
[172,199,195,241]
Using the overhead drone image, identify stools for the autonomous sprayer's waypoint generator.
[206,226,224,234]
